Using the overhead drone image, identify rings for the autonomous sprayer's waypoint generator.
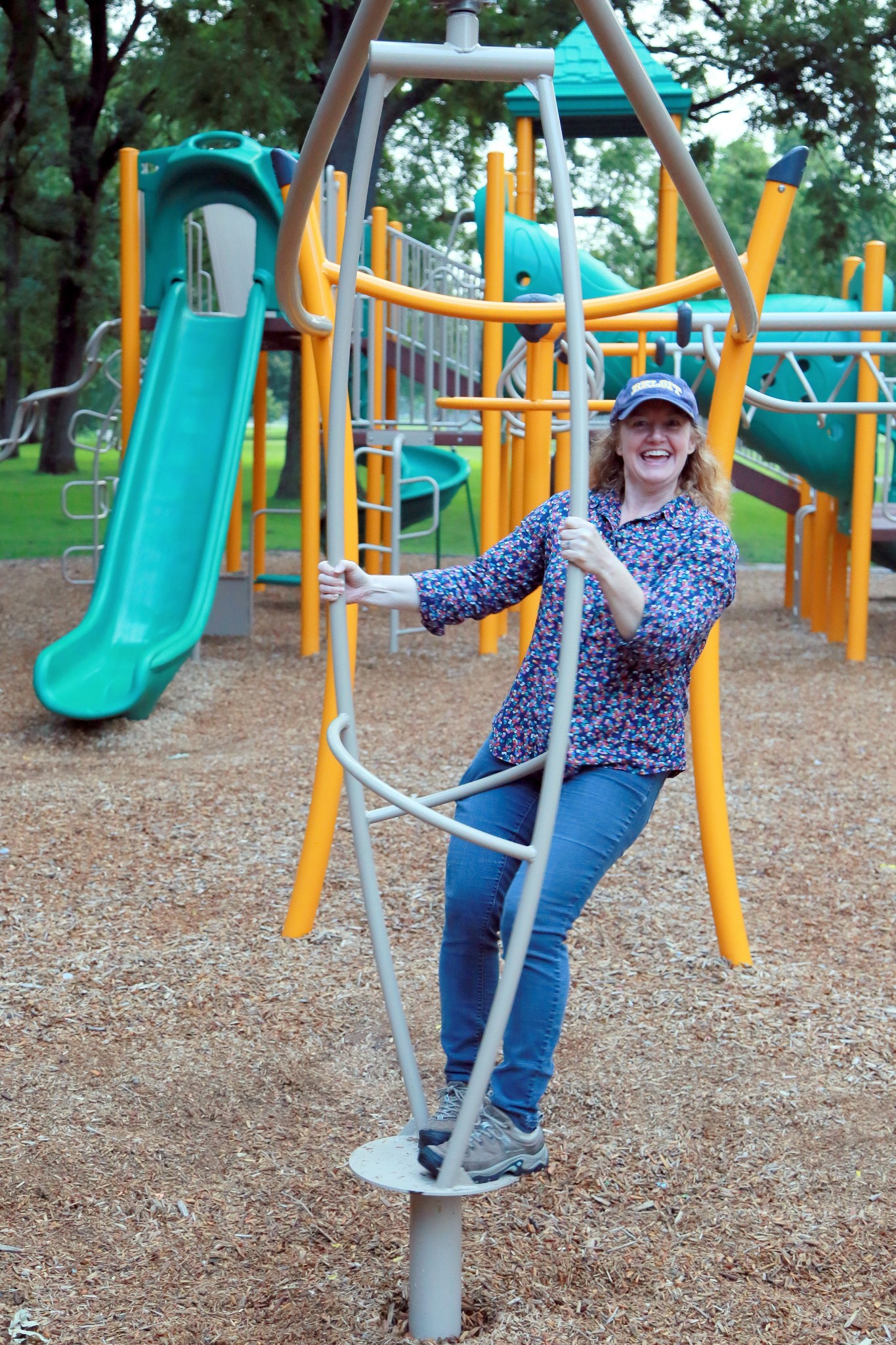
[566,540,571,550]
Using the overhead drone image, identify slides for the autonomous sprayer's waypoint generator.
[502,209,862,497]
[319,444,472,569]
[33,278,270,721]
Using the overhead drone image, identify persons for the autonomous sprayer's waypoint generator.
[318,372,738,1184]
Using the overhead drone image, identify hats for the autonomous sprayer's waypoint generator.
[609,373,699,428]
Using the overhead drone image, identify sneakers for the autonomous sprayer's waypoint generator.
[419,1081,490,1142]
[418,1105,551,1183]
